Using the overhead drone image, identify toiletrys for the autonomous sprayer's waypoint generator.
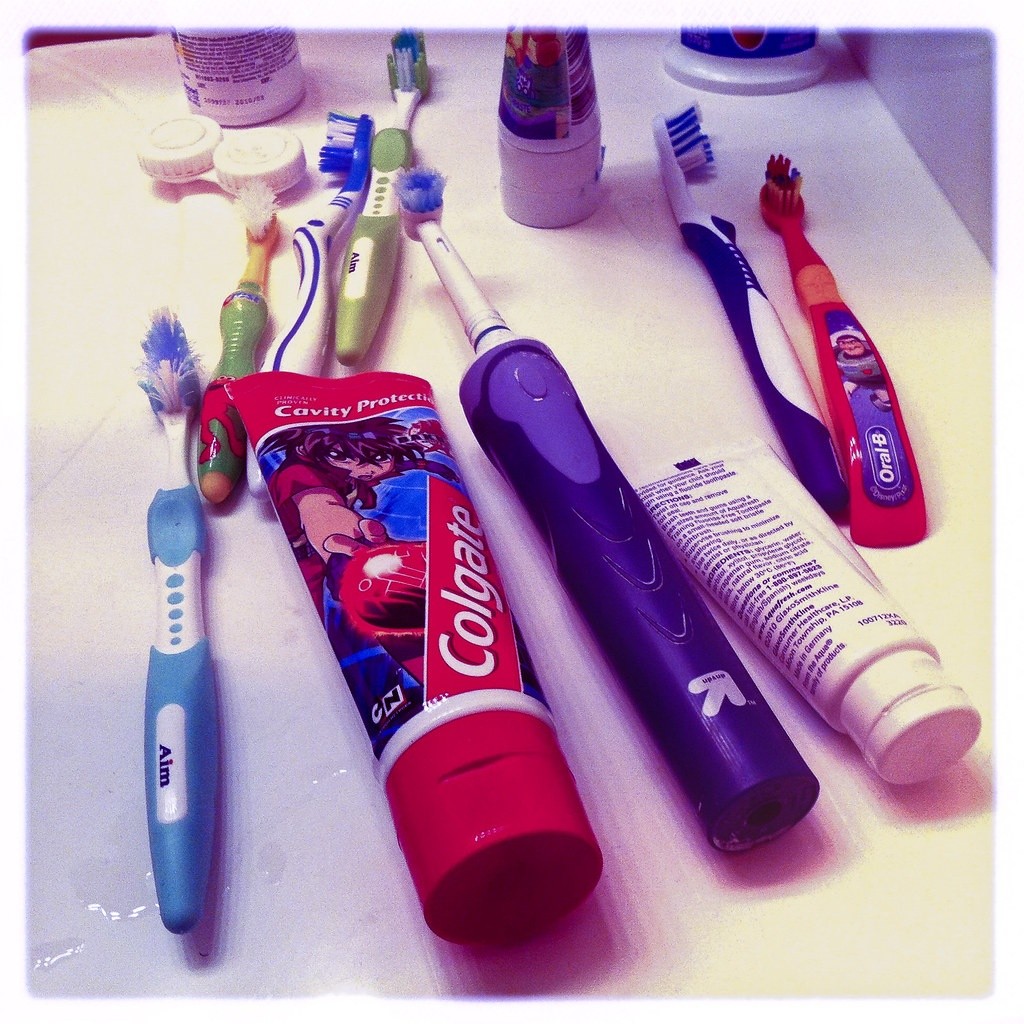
[497,29,605,232]
[216,371,621,948]
[165,28,307,129]
[627,435,983,786]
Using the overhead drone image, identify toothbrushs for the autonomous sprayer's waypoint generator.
[334,27,434,363]
[756,151,930,549]
[650,104,850,515]
[392,162,821,856]
[133,304,222,937]
[196,180,283,507]
[256,107,374,376]
